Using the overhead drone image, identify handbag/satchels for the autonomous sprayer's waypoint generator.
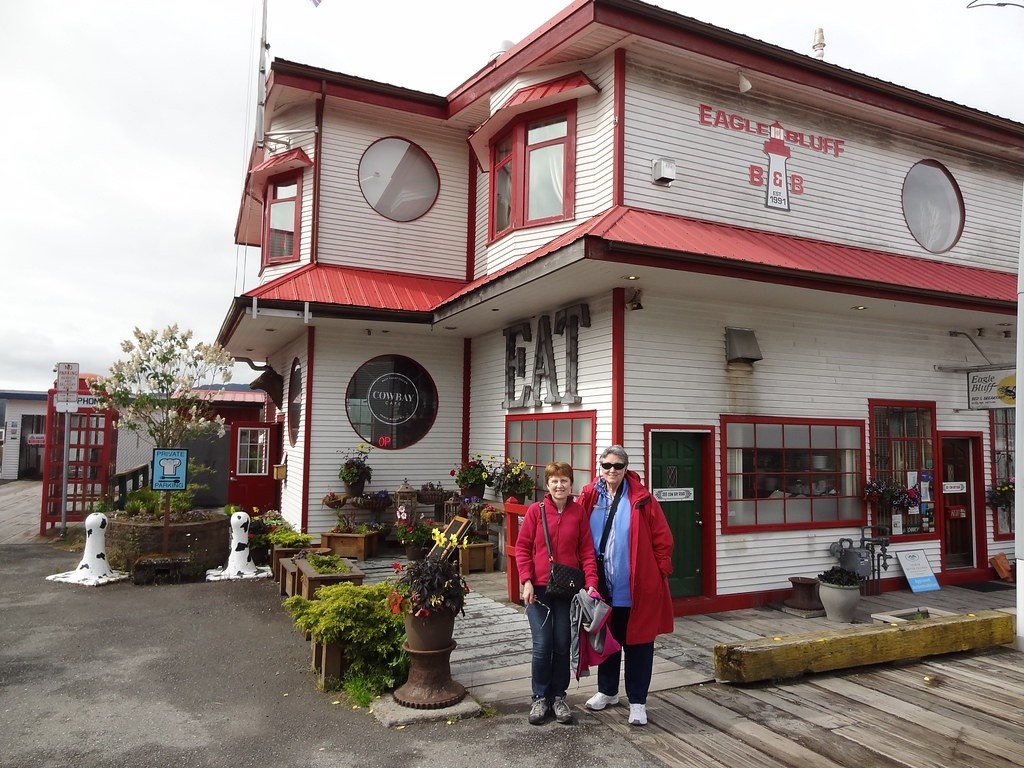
[545,563,585,601]
[596,555,612,603]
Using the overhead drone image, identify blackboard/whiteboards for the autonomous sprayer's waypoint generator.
[426,515,470,563]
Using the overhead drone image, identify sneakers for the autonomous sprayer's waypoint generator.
[528,697,550,724]
[586,691,620,710]
[628,702,648,723]
[550,695,573,722]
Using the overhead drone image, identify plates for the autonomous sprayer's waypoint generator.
[800,456,827,468]
[820,469,833,472]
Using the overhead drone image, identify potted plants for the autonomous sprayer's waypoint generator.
[415,480,449,506]
[818,566,865,623]
[266,527,313,582]
[281,577,410,692]
[278,548,319,600]
[326,497,346,509]
[871,606,958,625]
[295,552,366,601]
[320,506,379,562]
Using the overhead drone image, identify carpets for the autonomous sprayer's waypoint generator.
[947,580,1016,593]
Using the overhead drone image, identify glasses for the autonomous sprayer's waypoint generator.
[600,461,626,470]
[522,598,551,629]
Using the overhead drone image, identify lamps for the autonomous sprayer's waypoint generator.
[273,464,286,480]
[978,328,987,337]
[1003,331,1011,339]
[735,66,751,93]
[651,157,676,188]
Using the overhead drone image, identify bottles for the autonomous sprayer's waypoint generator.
[922,511,935,533]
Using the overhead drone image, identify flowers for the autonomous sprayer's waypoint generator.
[336,444,375,486]
[394,506,437,545]
[320,485,348,509]
[387,528,470,623]
[362,488,391,500]
[449,453,539,525]
[863,478,922,507]
[987,477,1015,505]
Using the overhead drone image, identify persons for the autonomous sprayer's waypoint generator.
[574,446,675,725]
[516,464,600,726]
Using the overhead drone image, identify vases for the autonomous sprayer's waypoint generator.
[392,637,467,710]
[402,609,455,651]
[461,483,484,500]
[404,542,424,561]
[344,478,366,496]
[783,576,822,610]
[502,491,525,507]
[351,497,392,510]
[813,455,828,469]
[461,507,488,530]
